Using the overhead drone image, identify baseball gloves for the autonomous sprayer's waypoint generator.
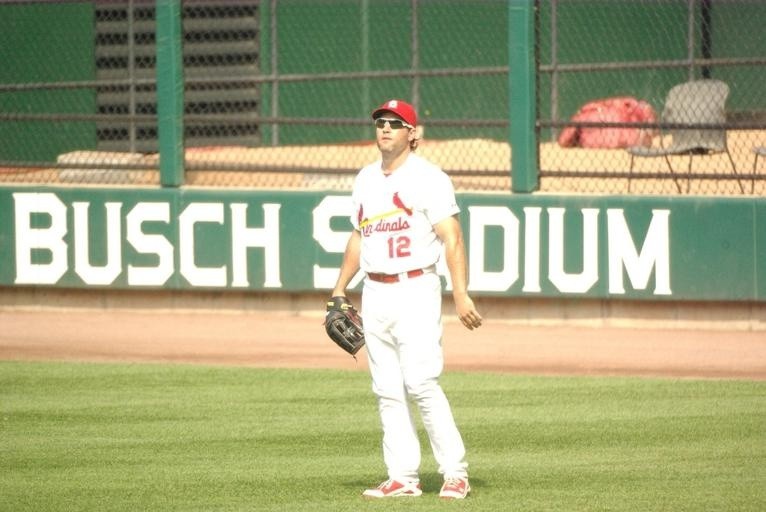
[325,297,366,354]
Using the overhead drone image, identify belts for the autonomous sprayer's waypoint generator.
[367,269,423,283]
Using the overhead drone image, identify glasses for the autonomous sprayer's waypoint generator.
[374,116,414,130]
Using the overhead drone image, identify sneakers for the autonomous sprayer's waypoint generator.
[363,479,423,497]
[439,478,470,499]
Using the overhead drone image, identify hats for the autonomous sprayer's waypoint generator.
[371,99,416,129]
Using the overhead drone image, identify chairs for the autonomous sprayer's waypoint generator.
[627,78,766,194]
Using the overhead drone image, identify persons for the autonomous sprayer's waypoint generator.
[326,100,484,500]
